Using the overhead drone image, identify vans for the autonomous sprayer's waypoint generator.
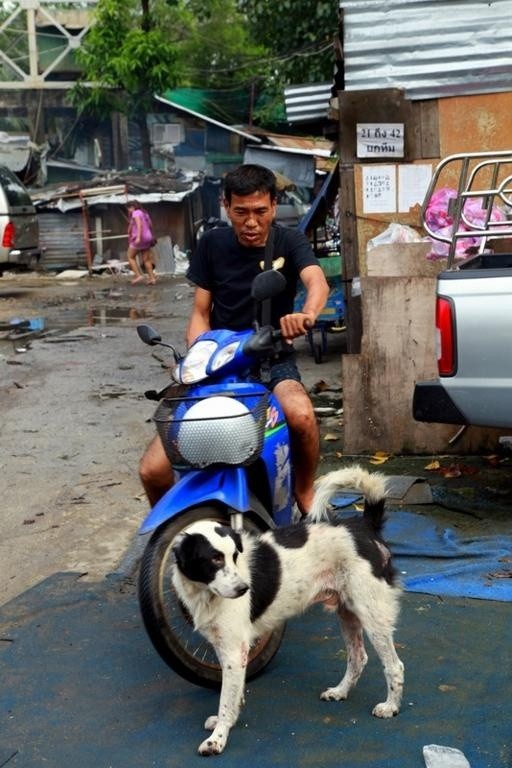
[0,159,43,277]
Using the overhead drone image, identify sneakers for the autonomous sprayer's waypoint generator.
[146,278,156,286]
[130,275,144,286]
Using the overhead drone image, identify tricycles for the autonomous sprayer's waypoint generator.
[297,231,345,366]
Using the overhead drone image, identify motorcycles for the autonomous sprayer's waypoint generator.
[124,269,327,692]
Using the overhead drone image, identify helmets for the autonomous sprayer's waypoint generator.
[172,395,261,470]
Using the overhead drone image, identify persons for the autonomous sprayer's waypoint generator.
[136,161,332,530]
[124,200,157,288]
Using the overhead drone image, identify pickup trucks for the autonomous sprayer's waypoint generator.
[405,143,512,439]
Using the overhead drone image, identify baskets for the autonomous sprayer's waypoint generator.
[148,390,273,472]
[317,255,343,278]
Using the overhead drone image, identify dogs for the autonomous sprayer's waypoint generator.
[170,464,405,757]
